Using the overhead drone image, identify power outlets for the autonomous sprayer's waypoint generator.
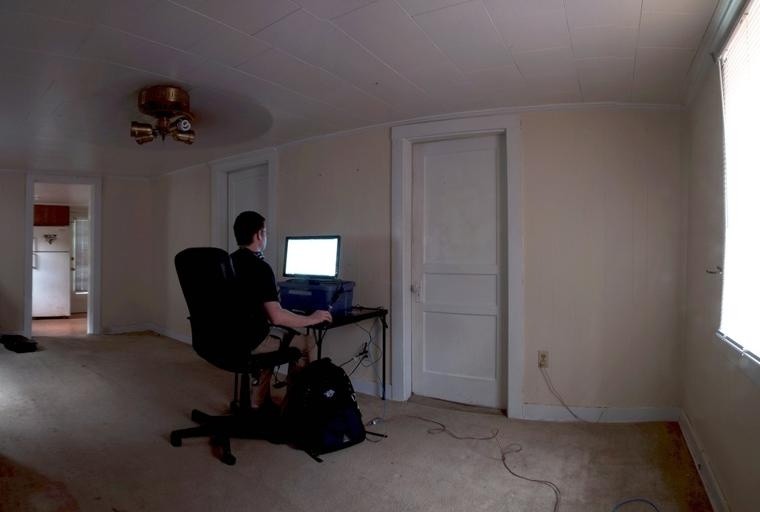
[537,350,549,369]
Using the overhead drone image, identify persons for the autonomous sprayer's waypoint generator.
[228,209,334,410]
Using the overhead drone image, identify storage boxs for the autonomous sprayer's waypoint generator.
[277,278,356,316]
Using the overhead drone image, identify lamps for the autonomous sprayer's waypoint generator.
[128,83,199,146]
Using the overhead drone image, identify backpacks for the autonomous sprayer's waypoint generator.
[282,357,368,457]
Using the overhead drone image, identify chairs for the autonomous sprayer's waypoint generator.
[168,245,302,467]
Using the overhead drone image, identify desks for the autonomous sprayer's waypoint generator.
[271,306,389,402]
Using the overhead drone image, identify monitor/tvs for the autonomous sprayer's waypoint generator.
[283,234,342,285]
